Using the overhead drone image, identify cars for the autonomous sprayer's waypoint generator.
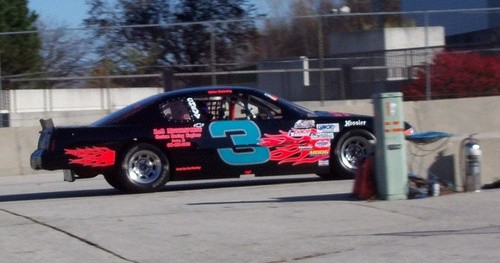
[29,85,415,192]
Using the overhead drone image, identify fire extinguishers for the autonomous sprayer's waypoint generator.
[462,133,482,193]
